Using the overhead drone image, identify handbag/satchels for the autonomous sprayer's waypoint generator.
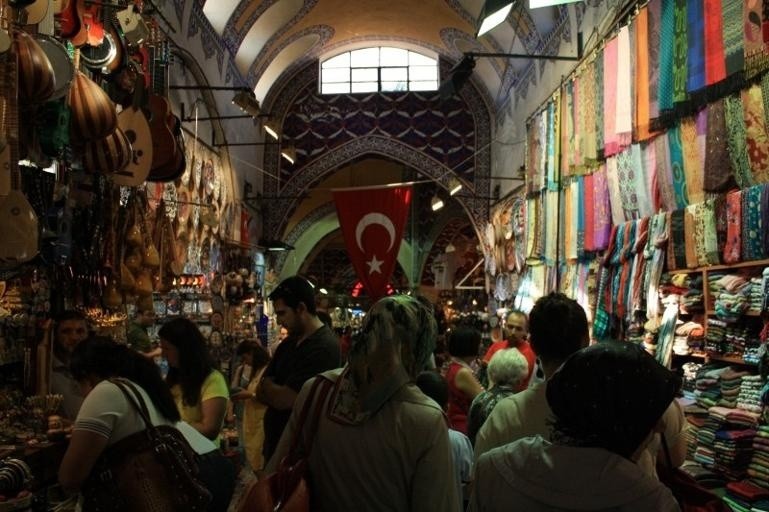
[224,374,340,512]
[87,374,215,512]
[653,428,733,512]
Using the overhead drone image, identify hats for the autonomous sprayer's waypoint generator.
[542,339,685,459]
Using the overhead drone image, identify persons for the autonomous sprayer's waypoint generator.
[417,294,687,512]
[256,277,356,463]
[265,296,463,512]
[48,310,271,511]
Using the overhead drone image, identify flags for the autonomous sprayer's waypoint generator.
[331,181,411,304]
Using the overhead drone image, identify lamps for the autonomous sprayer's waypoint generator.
[175,100,286,141]
[443,171,527,198]
[434,29,603,99]
[168,78,258,119]
[471,0,522,44]
[425,184,502,212]
[261,235,295,255]
[207,131,302,168]
